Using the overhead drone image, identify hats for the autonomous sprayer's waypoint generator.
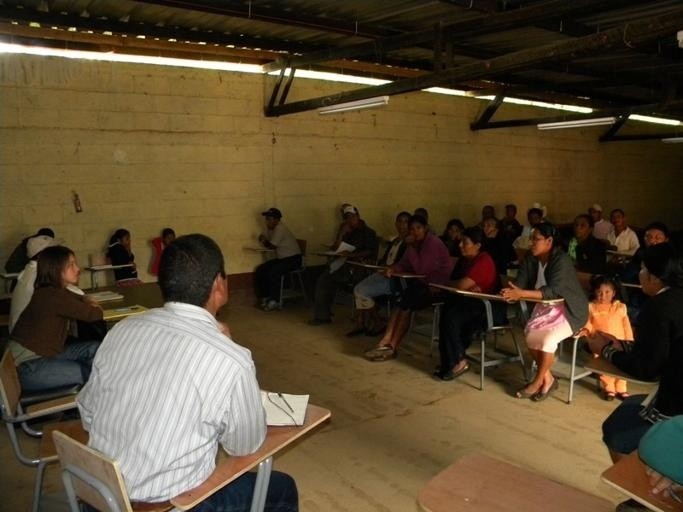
[588,204,602,212]
[26,235,59,259]
[262,208,282,218]
[343,206,358,219]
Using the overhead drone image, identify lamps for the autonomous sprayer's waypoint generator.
[663,137,680,144]
[318,95,390,115]
[537,117,616,130]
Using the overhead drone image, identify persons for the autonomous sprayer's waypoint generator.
[572,278,635,401]
[306,202,451,361]
[638,415,682,498]
[585,243,683,464]
[151,228,176,276]
[499,222,590,398]
[75,233,298,512]
[415,205,667,353]
[3,228,109,393]
[109,228,144,288]
[254,208,304,311]
[433,227,498,380]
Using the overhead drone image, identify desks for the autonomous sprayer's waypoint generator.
[600,449,683,512]
[417,449,616,512]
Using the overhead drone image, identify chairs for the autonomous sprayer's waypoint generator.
[240,236,661,404]
[54,391,332,512]
[1,236,150,511]
